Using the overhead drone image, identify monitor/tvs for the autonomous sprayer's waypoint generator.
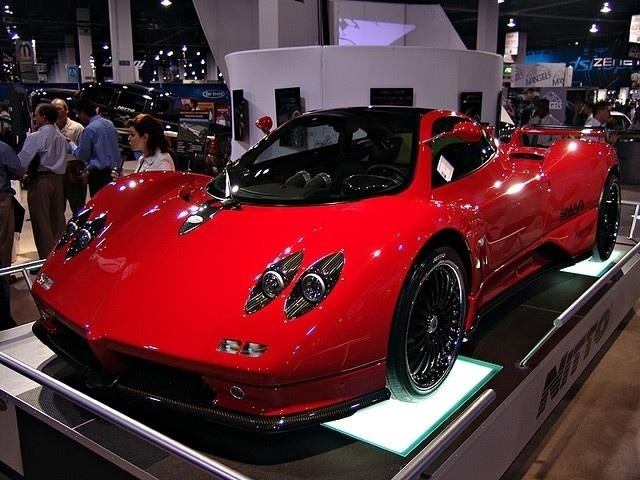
[274,86,301,147]
[496,91,503,137]
[369,87,414,107]
[232,89,249,141]
[460,91,482,121]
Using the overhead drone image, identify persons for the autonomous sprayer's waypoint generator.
[0,100,32,155]
[188,97,200,111]
[66,95,122,198]
[18,102,67,275]
[110,112,175,182]
[0,138,26,332]
[498,88,640,153]
[51,99,89,218]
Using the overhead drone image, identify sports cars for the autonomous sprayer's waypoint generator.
[30,100,625,436]
